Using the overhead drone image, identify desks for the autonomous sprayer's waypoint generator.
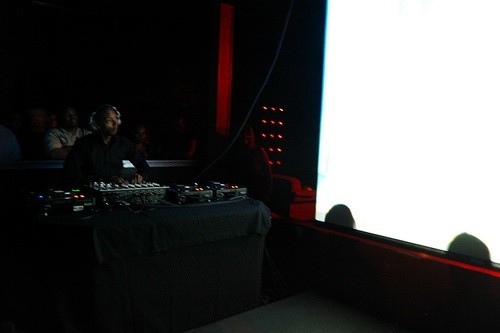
[40,197,272,333]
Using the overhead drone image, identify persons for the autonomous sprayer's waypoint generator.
[67,105,143,186]
[1,107,203,168]
[49,109,93,157]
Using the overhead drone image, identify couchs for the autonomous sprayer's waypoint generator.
[272,172,316,222]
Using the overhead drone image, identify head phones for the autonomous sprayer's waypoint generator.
[89,104,122,131]
